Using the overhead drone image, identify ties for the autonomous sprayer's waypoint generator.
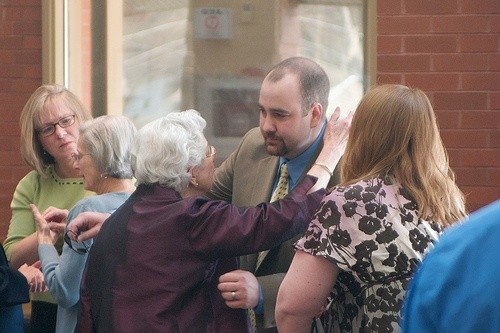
[247,163,289,333]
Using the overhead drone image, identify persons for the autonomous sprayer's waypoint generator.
[30,114,138,333]
[3,84,94,333]
[73,110,355,333]
[68,57,341,333]
[275,83,469,333]
[0,241,47,333]
[400,199,500,333]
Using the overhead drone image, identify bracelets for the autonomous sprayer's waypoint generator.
[314,162,333,176]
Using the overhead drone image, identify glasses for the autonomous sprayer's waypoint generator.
[206,145,217,160]
[63,229,90,255]
[71,152,91,161]
[38,114,76,136]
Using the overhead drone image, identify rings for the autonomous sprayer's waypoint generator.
[232,292,236,300]
